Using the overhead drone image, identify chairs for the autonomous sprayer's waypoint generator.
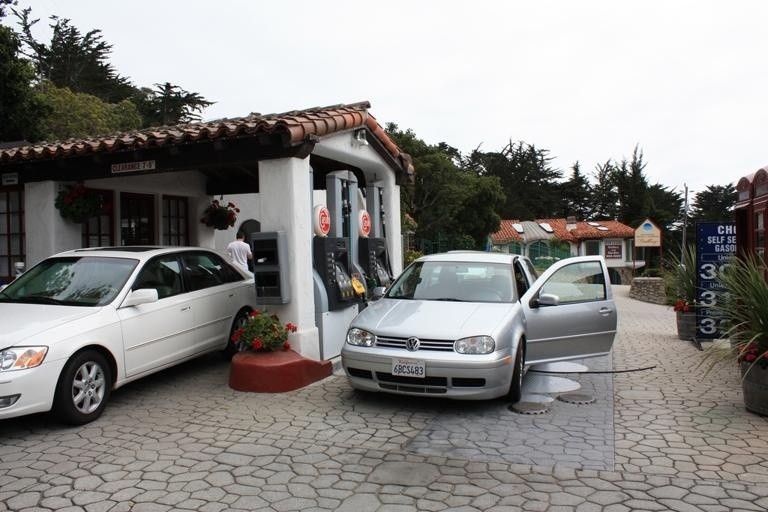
[484,272,512,300]
[425,268,463,298]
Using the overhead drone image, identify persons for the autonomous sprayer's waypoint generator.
[225,230,253,272]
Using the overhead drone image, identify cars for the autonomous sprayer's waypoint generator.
[0,245,258,424]
[341,250,617,403]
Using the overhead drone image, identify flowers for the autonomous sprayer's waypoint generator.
[230,309,298,353]
[683,246,768,387]
[659,238,696,313]
[200,199,240,230]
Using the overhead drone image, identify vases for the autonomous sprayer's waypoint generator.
[740,361,768,417]
[676,310,696,341]
